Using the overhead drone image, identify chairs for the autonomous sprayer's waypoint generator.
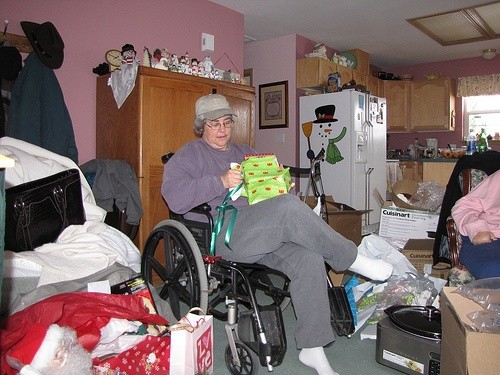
[432,150,500,286]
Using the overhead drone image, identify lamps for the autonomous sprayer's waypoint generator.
[481,48,496,60]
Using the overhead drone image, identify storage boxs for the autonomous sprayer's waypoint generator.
[370,188,441,274]
[299,195,374,247]
[440,286,500,375]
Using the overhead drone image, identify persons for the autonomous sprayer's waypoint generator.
[160,93,393,375]
[120,44,223,80]
[450,169,500,289]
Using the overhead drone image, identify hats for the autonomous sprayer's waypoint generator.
[195,93,238,121]
[0,46,23,80]
[19,21,65,69]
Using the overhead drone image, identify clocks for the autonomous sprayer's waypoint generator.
[105,49,122,68]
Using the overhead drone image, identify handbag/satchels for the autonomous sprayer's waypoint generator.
[169,307,213,375]
[4,168,87,253]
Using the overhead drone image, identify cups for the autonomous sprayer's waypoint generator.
[425,138,438,159]
[448,143,456,152]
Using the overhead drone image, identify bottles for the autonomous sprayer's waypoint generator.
[466,129,477,155]
[477,128,488,153]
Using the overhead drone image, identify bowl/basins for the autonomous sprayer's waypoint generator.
[398,73,414,80]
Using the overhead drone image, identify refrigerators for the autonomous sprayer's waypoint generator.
[299,89,387,237]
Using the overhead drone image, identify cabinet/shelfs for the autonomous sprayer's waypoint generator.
[423,160,457,188]
[95,66,256,286]
[296,56,455,132]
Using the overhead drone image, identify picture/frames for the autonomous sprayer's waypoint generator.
[259,80,289,129]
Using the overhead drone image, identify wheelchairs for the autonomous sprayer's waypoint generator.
[141,151,356,375]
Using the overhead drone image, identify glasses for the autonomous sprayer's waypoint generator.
[205,120,235,129]
